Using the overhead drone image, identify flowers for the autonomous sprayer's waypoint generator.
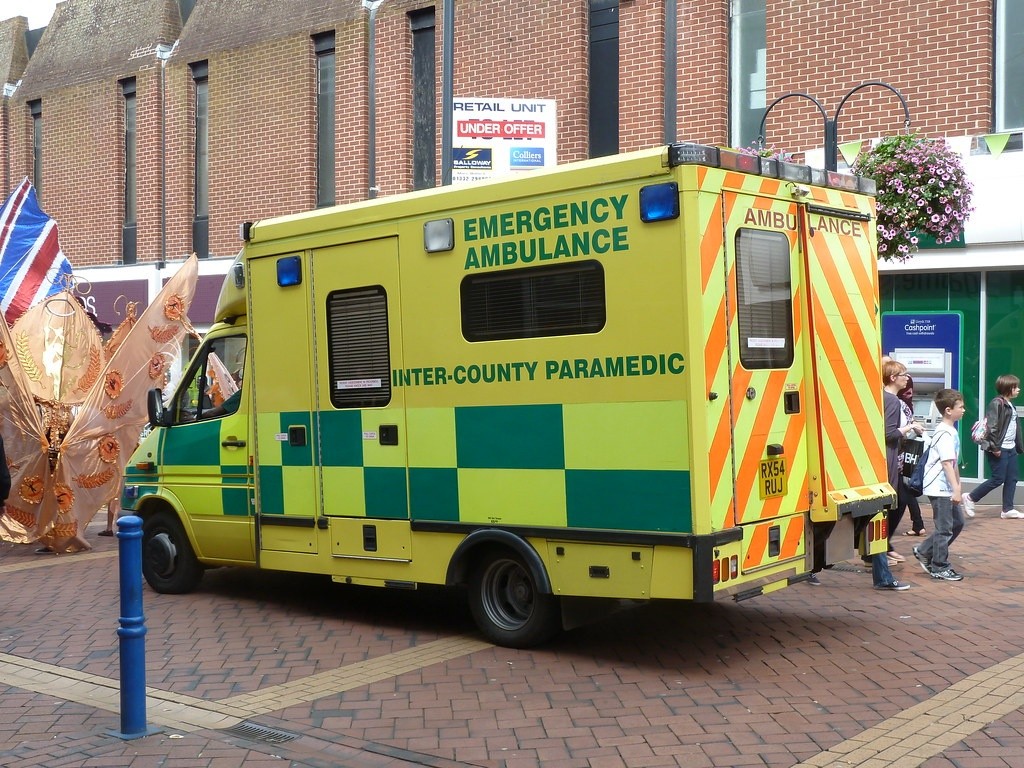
[735,141,793,163]
[851,126,978,265]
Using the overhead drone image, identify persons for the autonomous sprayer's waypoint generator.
[912,389,964,582]
[808,361,926,592]
[35,406,93,556]
[179,374,242,421]
[96,496,122,538]
[961,374,1024,519]
[0,434,12,519]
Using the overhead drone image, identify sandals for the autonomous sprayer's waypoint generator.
[902,527,927,537]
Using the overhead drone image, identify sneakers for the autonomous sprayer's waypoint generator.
[913,546,931,573]
[1000,508,1024,519]
[807,572,820,585]
[874,578,911,590]
[930,567,964,580]
[961,492,975,518]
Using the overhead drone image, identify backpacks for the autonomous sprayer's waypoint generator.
[908,430,951,497]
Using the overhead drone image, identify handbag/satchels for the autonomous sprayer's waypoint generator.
[971,398,1004,444]
[901,434,924,479]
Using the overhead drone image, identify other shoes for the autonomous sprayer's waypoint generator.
[886,551,905,561]
[864,556,897,567]
[98,529,113,536]
[34,546,56,553]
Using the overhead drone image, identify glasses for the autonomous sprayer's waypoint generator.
[894,372,907,378]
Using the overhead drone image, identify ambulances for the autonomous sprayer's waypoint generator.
[117,138,901,651]
